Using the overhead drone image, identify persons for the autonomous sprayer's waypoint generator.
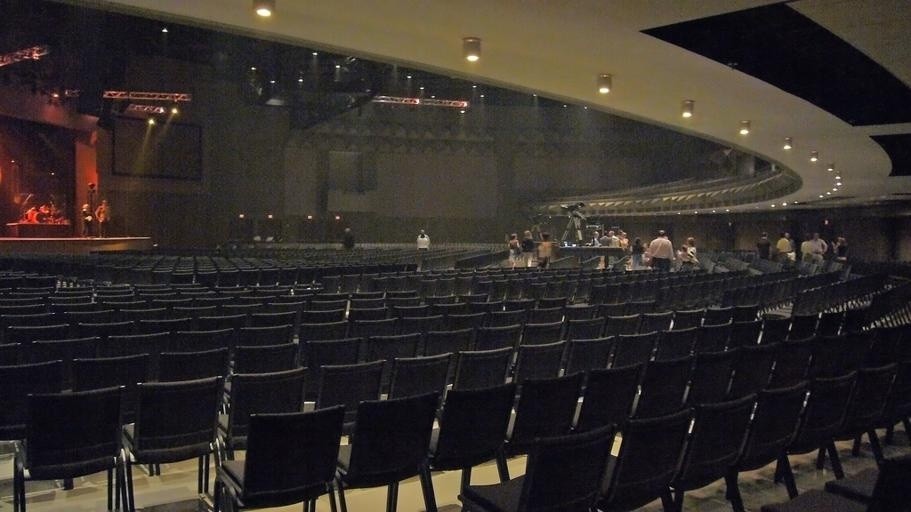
[522,230,536,265]
[415,229,431,251]
[756,233,771,260]
[341,227,356,249]
[589,227,700,273]
[508,234,522,266]
[78,199,114,237]
[776,233,848,272]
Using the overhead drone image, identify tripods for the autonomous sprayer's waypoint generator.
[562,217,585,243]
